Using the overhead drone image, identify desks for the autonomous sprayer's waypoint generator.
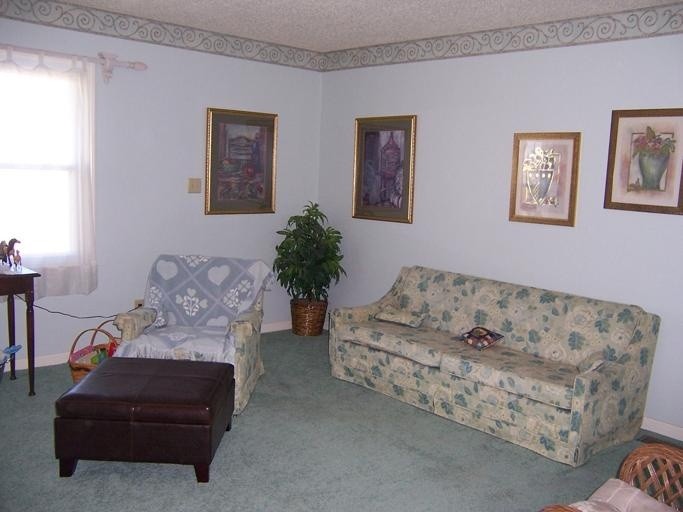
[0,262,42,396]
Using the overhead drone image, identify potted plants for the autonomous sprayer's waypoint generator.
[272,201,348,336]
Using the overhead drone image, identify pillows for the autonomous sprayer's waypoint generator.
[373,305,425,329]
[452,326,504,351]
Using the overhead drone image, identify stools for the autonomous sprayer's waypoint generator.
[53,356,236,483]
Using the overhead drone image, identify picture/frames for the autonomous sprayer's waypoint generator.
[353,114,417,224]
[603,107,683,215]
[206,108,278,215]
[508,133,581,226]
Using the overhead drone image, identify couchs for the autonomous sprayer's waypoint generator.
[113,255,272,417]
[535,443,683,512]
[326,263,660,468]
